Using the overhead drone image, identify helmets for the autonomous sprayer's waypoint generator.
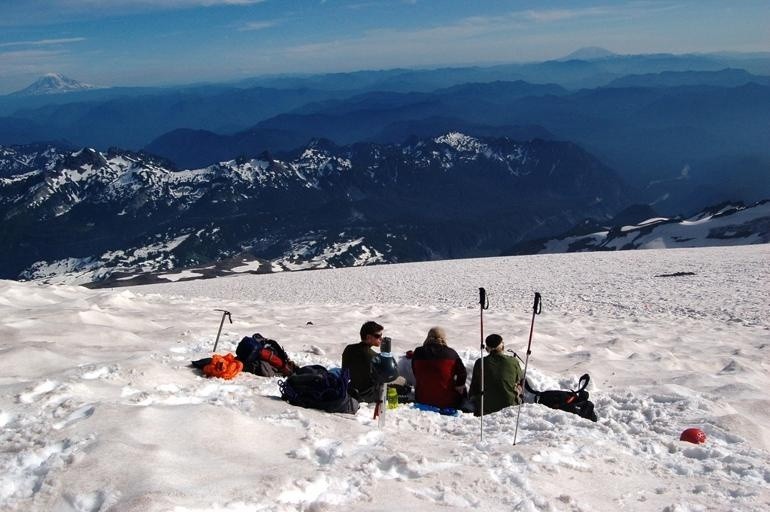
[680,428,706,444]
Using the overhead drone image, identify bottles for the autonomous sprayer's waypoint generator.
[388,387,398,408]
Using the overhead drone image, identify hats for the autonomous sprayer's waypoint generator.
[486,334,502,347]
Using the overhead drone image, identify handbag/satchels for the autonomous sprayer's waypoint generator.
[535,388,597,422]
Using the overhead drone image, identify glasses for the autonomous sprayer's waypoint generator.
[371,333,383,338]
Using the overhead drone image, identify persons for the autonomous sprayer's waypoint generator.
[340,320,411,403]
[468,333,525,416]
[412,326,467,409]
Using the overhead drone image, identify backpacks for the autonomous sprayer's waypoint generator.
[234,333,287,372]
